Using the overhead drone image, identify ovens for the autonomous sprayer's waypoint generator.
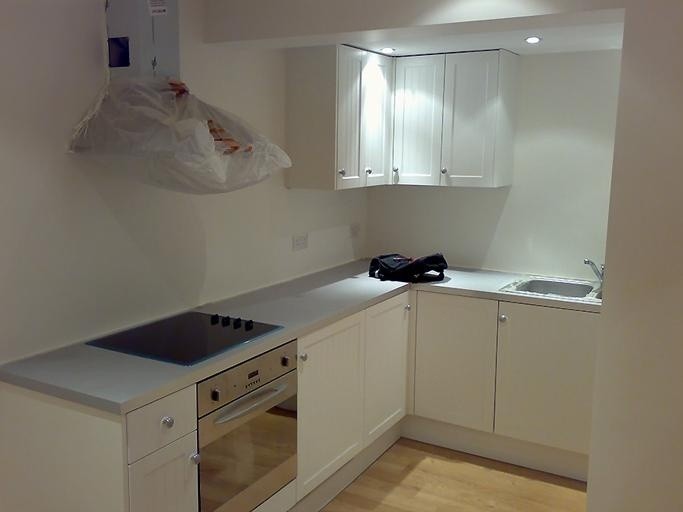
[195,338,298,511]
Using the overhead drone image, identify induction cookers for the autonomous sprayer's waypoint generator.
[84,311,283,366]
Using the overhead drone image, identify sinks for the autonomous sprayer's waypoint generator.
[500,274,602,306]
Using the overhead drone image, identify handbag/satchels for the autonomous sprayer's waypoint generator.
[369,253,448,284]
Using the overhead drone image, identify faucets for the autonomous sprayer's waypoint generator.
[583,259,604,282]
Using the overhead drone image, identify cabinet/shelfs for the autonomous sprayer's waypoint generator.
[405,284,603,482]
[297,282,411,512]
[393,49,522,189]
[282,44,396,190]
[0,380,199,512]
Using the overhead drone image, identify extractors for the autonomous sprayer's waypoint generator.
[71,0,242,159]
